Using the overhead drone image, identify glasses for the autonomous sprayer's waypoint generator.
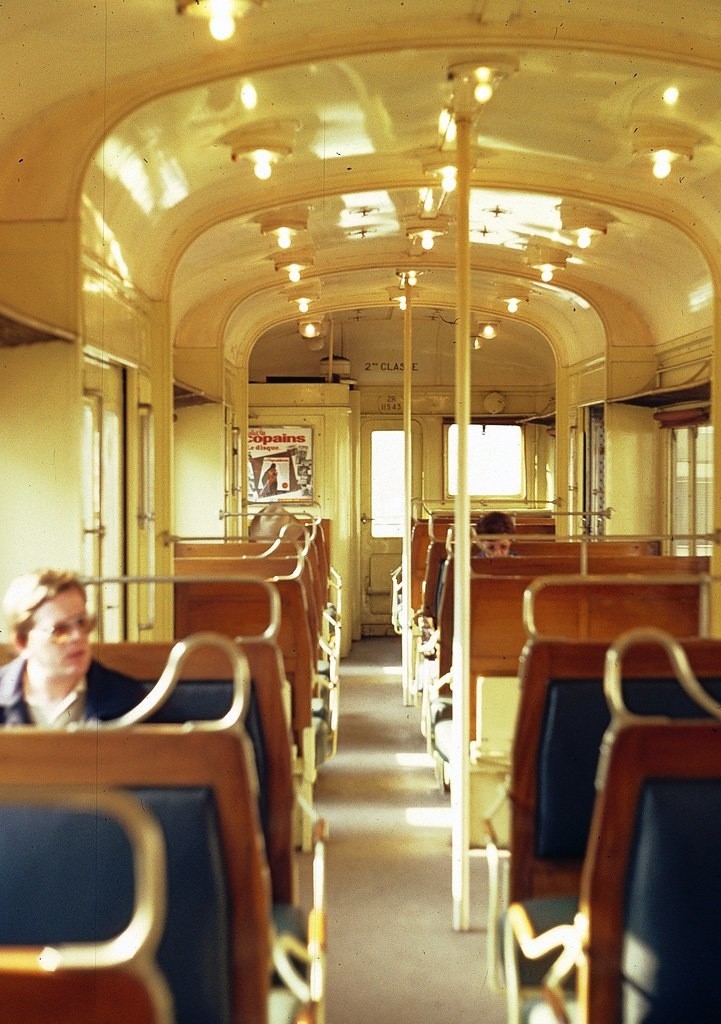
[482,542,508,551]
[27,614,97,648]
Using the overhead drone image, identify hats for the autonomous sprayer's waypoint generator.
[250,504,303,544]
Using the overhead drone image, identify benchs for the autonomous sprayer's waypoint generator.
[391,496,720,1024]
[0,502,343,1024]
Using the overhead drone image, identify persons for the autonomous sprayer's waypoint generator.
[473,511,517,558]
[0,568,186,728]
[249,503,304,544]
